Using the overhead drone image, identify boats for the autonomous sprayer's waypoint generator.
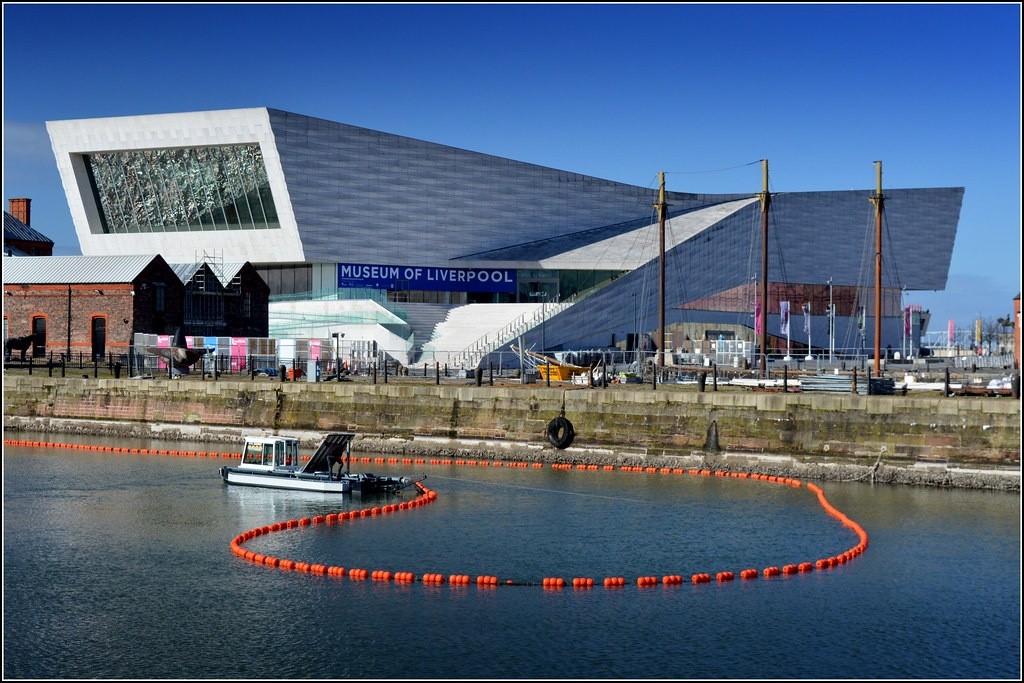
[222,432,411,498]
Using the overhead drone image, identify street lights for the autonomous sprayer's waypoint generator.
[331,331,345,382]
[827,276,834,364]
[751,273,759,347]
